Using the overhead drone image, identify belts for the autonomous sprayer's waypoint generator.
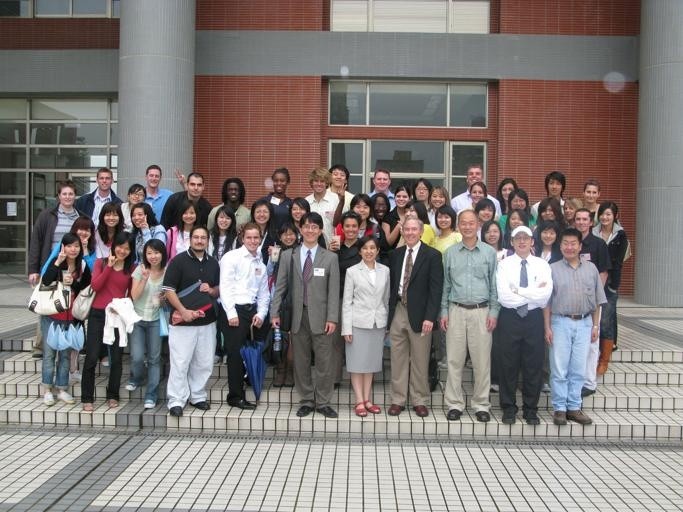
[560,311,592,321]
[451,300,488,309]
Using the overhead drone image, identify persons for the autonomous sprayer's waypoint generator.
[582,178,601,224]
[495,225,553,425]
[531,170,566,219]
[257,167,293,223]
[440,209,502,422]
[159,172,213,231]
[531,197,565,235]
[367,169,397,212]
[205,205,240,365]
[536,219,563,393]
[124,238,174,409]
[232,198,277,267]
[589,201,629,377]
[543,228,608,425]
[475,197,496,242]
[143,164,187,225]
[328,163,356,215]
[39,233,92,406]
[480,220,508,392]
[450,163,502,222]
[395,200,439,393]
[219,222,271,410]
[331,182,391,228]
[498,176,517,215]
[130,202,167,265]
[289,197,330,251]
[120,183,147,232]
[456,181,488,231]
[303,166,348,239]
[380,183,413,251]
[503,209,530,251]
[73,167,124,223]
[563,197,585,227]
[334,193,379,238]
[162,226,220,417]
[271,212,340,417]
[167,199,200,267]
[574,208,612,397]
[41,215,97,383]
[94,201,126,367]
[267,220,300,387]
[27,179,86,359]
[429,204,463,392]
[330,210,363,391]
[386,217,444,417]
[427,185,451,236]
[81,231,138,411]
[206,177,252,232]
[499,188,537,248]
[341,234,391,417]
[413,178,433,213]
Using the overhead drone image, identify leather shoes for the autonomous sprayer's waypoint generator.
[169,406,182,416]
[413,404,428,417]
[188,401,209,409]
[316,406,338,417]
[446,409,462,419]
[502,408,515,424]
[32,354,41,358]
[387,404,405,415]
[229,399,256,409]
[581,386,595,397]
[296,406,315,416]
[521,409,540,425]
[475,411,489,421]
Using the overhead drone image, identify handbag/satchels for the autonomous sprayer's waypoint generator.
[28,263,70,316]
[139,261,173,335]
[71,257,105,321]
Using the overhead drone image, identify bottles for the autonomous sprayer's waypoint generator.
[273,326,281,351]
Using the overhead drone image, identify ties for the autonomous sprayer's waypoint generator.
[302,249,312,307]
[519,260,528,287]
[400,249,413,307]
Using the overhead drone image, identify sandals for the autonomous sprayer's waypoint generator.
[364,400,381,414]
[82,402,92,410]
[354,403,367,416]
[106,398,117,407]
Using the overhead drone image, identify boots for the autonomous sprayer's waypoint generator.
[284,360,294,386]
[272,361,284,386]
[597,338,614,375]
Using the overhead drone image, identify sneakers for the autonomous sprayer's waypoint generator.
[490,383,498,391]
[566,409,592,424]
[43,391,54,405]
[57,390,74,404]
[68,370,81,383]
[124,382,136,390]
[143,399,155,408]
[541,383,552,391]
[554,410,566,425]
[100,361,109,366]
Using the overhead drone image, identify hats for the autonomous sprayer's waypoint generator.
[511,225,533,236]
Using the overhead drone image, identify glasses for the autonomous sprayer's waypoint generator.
[514,237,528,241]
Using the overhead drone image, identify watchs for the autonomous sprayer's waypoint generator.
[592,325,599,329]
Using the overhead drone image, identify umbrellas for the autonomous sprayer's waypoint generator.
[239,322,269,403]
